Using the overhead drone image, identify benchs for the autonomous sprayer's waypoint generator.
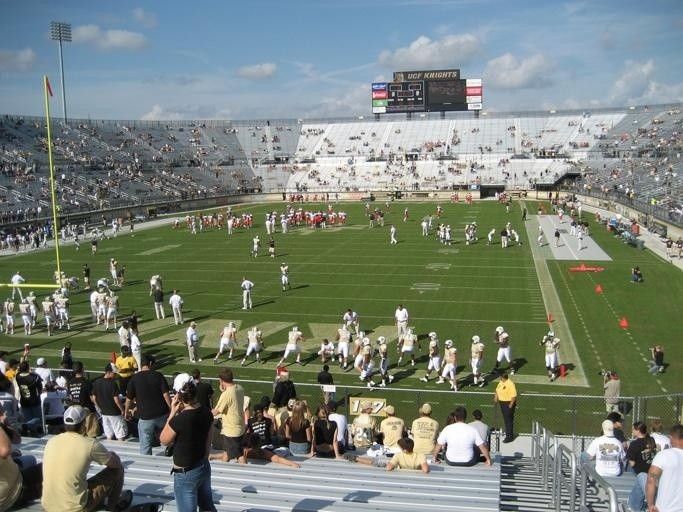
[0,117,260,217]
[568,103,680,220]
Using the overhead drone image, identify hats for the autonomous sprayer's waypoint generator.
[173,373,196,394]
[361,401,373,409]
[607,412,624,421]
[601,419,614,437]
[422,403,432,413]
[288,398,296,408]
[64,405,90,425]
[384,405,395,414]
[37,357,48,365]
[105,362,121,373]
[191,321,197,326]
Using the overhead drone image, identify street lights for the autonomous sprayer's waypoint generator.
[50,18,71,129]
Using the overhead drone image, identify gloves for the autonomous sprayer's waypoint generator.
[542,336,548,343]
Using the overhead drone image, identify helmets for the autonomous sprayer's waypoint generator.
[472,335,480,343]
[293,326,300,332]
[444,339,453,349]
[362,337,371,346]
[376,336,386,345]
[252,326,259,332]
[229,322,235,327]
[428,331,437,338]
[547,331,554,337]
[495,326,504,335]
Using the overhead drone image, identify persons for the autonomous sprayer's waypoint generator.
[647,345,664,375]
[632,266,643,283]
[2,354,517,512]
[1,108,683,256]
[541,330,561,381]
[1,252,515,404]
[584,412,683,511]
[604,371,622,416]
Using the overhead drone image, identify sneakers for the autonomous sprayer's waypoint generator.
[388,375,394,384]
[435,379,444,383]
[419,377,428,382]
[377,384,386,387]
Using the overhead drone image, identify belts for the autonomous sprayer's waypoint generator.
[170,467,189,475]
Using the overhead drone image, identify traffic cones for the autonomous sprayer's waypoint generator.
[237,205,239,208]
[541,205,543,210]
[560,365,565,376]
[547,312,552,322]
[596,284,603,292]
[620,317,628,328]
